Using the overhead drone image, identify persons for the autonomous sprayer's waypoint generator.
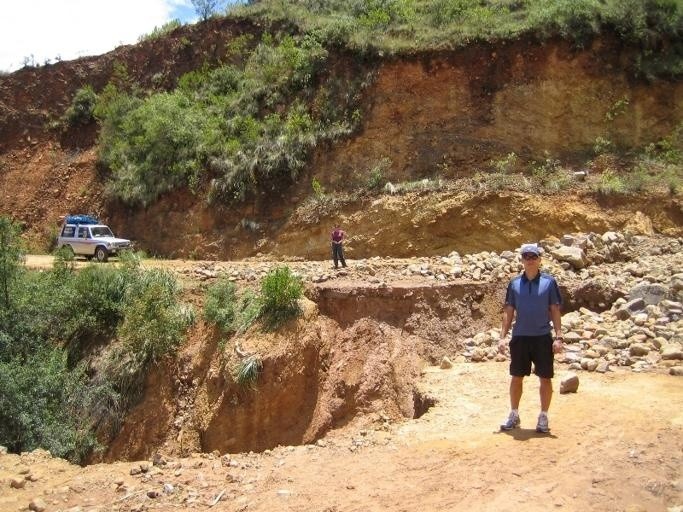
[496,243,565,433]
[330,222,349,269]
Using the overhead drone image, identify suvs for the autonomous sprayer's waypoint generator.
[57,214,132,262]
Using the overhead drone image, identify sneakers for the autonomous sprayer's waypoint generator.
[534,414,549,434]
[500,412,523,431]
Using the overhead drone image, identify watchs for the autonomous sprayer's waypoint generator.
[554,335,564,342]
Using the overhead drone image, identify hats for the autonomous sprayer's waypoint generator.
[521,245,541,257]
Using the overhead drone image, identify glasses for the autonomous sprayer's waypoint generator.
[523,255,539,260]
